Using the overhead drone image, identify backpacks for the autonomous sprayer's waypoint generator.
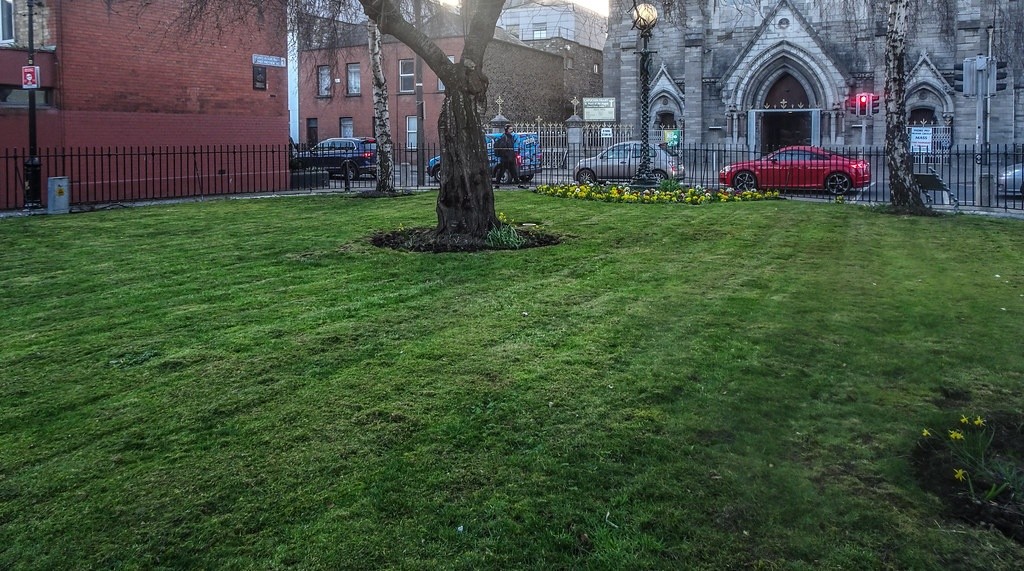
[494,137,505,155]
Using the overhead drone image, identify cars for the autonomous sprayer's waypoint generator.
[295,137,378,180]
[427,134,542,182]
[573,141,684,187]
[718,145,871,197]
[995,162,1024,197]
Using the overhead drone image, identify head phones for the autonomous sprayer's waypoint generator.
[504,124,509,132]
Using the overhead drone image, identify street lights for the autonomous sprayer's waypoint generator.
[630,4,660,187]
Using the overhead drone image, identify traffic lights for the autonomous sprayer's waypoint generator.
[850,93,880,116]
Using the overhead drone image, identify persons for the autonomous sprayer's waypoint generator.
[494,124,529,190]
